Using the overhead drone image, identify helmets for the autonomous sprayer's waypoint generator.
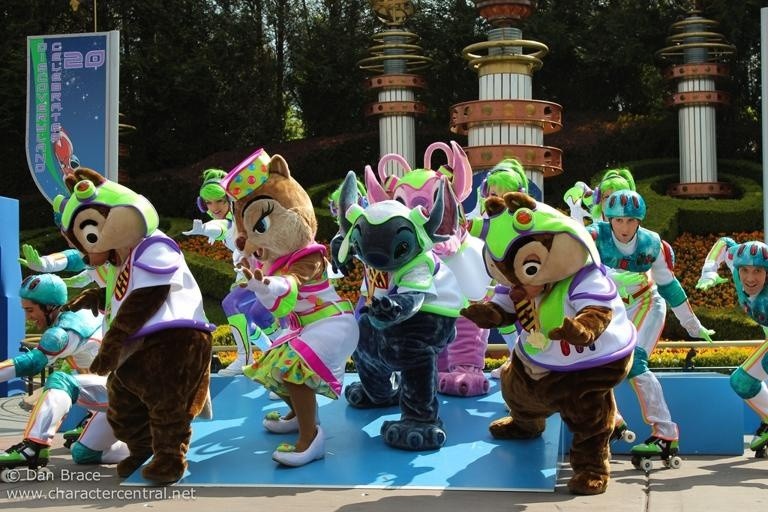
[734,241,767,269]
[19,274,69,306]
[604,190,647,221]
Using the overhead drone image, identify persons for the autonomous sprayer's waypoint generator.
[693,234,768,450]
[16,243,85,275]
[580,192,717,457]
[0,273,130,466]
[56,140,638,495]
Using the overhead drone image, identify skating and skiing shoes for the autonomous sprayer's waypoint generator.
[631,436,682,476]
[608,410,636,447]
[62,411,94,449]
[751,416,768,457]
[1,440,50,484]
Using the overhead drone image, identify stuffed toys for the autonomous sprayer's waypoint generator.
[56,139,639,496]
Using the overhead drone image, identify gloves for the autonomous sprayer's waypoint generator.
[183,219,208,236]
[696,273,729,292]
[689,328,715,343]
[17,244,46,274]
